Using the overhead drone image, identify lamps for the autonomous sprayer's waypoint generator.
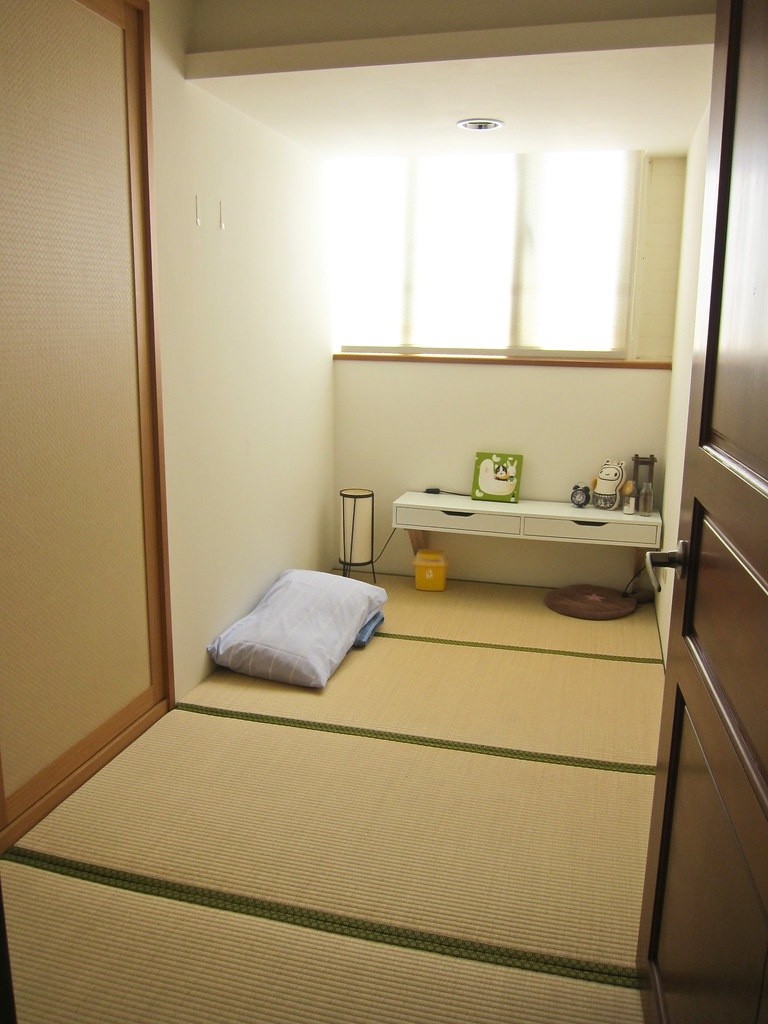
[338,488,378,584]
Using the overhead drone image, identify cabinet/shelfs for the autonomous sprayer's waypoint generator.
[391,491,662,593]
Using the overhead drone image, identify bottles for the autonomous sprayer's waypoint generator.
[623,481,637,514]
[638,482,654,516]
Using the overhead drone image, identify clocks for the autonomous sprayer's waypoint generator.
[571,481,591,508]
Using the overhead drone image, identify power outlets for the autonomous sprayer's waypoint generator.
[662,569,666,584]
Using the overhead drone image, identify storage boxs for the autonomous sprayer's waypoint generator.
[471,451,524,504]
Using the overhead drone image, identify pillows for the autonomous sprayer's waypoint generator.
[204,568,387,692]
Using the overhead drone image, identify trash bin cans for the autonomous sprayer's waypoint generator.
[411,548,449,591]
